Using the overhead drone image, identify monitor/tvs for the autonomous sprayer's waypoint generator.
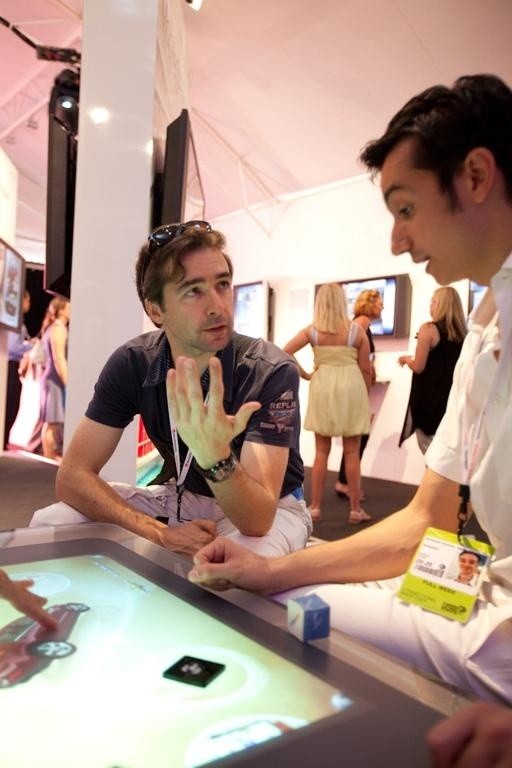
[42,116,73,299]
[159,110,207,229]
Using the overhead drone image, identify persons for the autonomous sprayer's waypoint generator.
[187,77,511,766]
[335,288,385,501]
[1,291,71,460]
[28,223,314,561]
[0,568,59,632]
[451,550,482,588]
[282,284,373,526]
[399,287,469,529]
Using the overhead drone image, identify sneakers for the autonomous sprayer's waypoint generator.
[348,508,372,525]
[306,505,321,522]
[335,480,367,502]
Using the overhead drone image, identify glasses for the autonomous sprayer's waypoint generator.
[139,219,214,286]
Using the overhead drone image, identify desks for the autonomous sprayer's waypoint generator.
[0,514,483,768]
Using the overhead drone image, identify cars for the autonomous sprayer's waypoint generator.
[0,601,90,687]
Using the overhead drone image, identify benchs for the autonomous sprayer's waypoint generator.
[273,528,401,610]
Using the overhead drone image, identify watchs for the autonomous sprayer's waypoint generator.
[194,451,237,482]
